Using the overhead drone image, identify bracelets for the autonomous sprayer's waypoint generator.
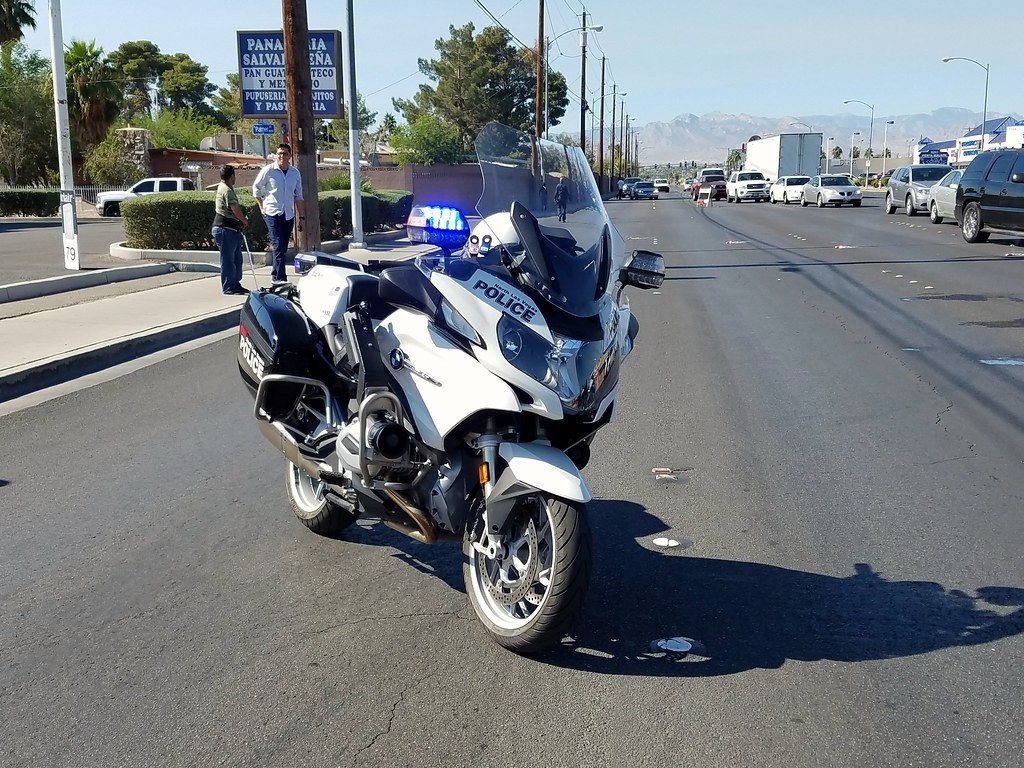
[299,216,304,220]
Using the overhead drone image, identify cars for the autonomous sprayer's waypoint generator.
[654,179,670,193]
[800,174,863,208]
[630,181,659,200]
[622,177,643,198]
[683,180,693,192]
[927,169,966,224]
[691,175,727,201]
[769,176,812,205]
[885,164,956,216]
[841,168,896,179]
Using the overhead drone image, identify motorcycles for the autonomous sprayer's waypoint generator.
[235,120,664,653]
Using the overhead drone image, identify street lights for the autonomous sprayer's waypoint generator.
[591,92,639,194]
[826,132,860,174]
[843,99,873,188]
[943,57,989,152]
[883,121,894,175]
[543,25,603,208]
[790,123,812,133]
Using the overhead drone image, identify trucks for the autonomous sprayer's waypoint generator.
[741,132,822,182]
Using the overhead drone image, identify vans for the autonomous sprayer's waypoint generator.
[697,168,724,175]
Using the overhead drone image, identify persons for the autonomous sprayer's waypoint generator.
[555,178,569,222]
[539,183,547,212]
[253,143,305,285]
[213,164,250,295]
[616,178,624,201]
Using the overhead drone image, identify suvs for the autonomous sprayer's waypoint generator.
[954,149,1024,243]
[726,170,770,203]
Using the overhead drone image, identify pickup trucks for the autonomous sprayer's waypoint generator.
[96,177,214,218]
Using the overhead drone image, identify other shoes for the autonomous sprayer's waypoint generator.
[559,215,562,222]
[223,287,250,296]
[271,277,286,285]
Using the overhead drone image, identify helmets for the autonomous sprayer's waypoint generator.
[466,209,521,254]
